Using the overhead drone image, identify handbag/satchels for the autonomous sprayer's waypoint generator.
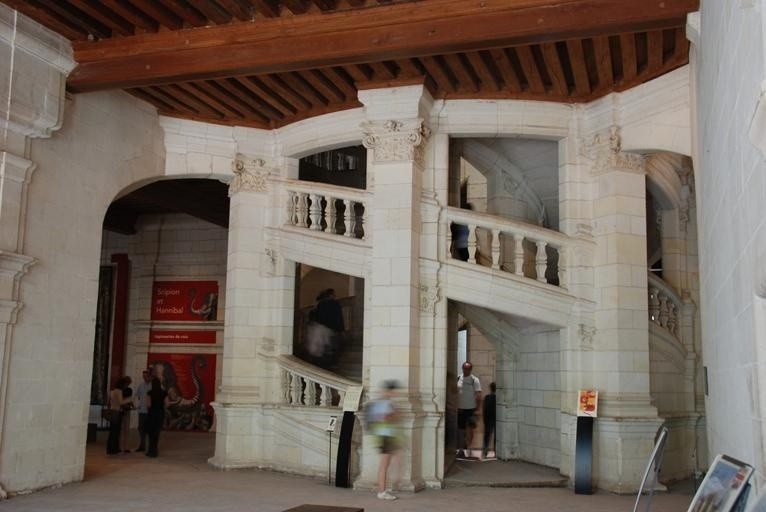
[102,409,120,423]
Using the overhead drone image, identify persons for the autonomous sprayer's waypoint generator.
[369,380,410,502]
[144,377,168,457]
[450,203,473,264]
[135,369,152,453]
[106,379,134,455]
[481,382,496,458]
[119,376,139,453]
[456,359,482,458]
[303,287,347,370]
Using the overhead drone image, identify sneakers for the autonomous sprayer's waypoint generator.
[377,489,397,500]
